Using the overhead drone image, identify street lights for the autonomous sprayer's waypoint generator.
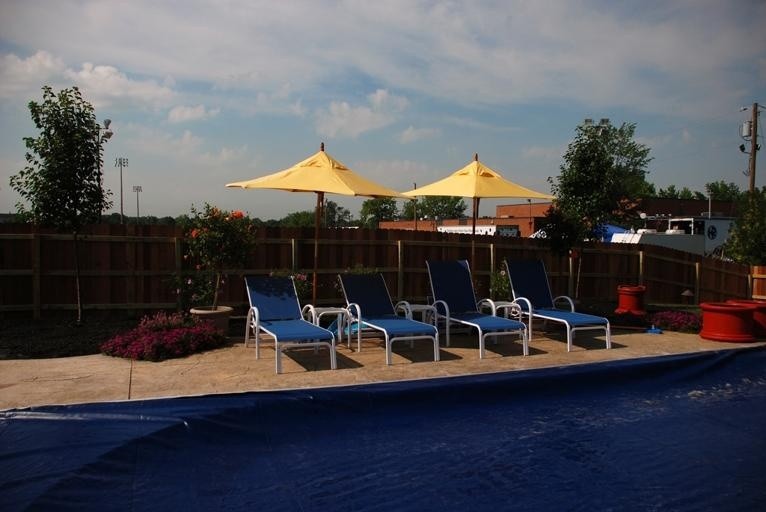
[94,118,114,225]
[115,158,129,225]
[132,186,142,227]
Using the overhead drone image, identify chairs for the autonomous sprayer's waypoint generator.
[425,259,530,360]
[243,275,338,372]
[505,258,611,353]
[337,273,443,366]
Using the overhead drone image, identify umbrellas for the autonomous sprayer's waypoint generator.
[402,152,560,292]
[225,142,417,306]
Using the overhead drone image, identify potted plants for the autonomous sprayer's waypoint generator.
[174,201,260,337]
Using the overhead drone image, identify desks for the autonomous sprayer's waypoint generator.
[481,302,520,346]
[397,304,437,349]
[307,306,353,352]
